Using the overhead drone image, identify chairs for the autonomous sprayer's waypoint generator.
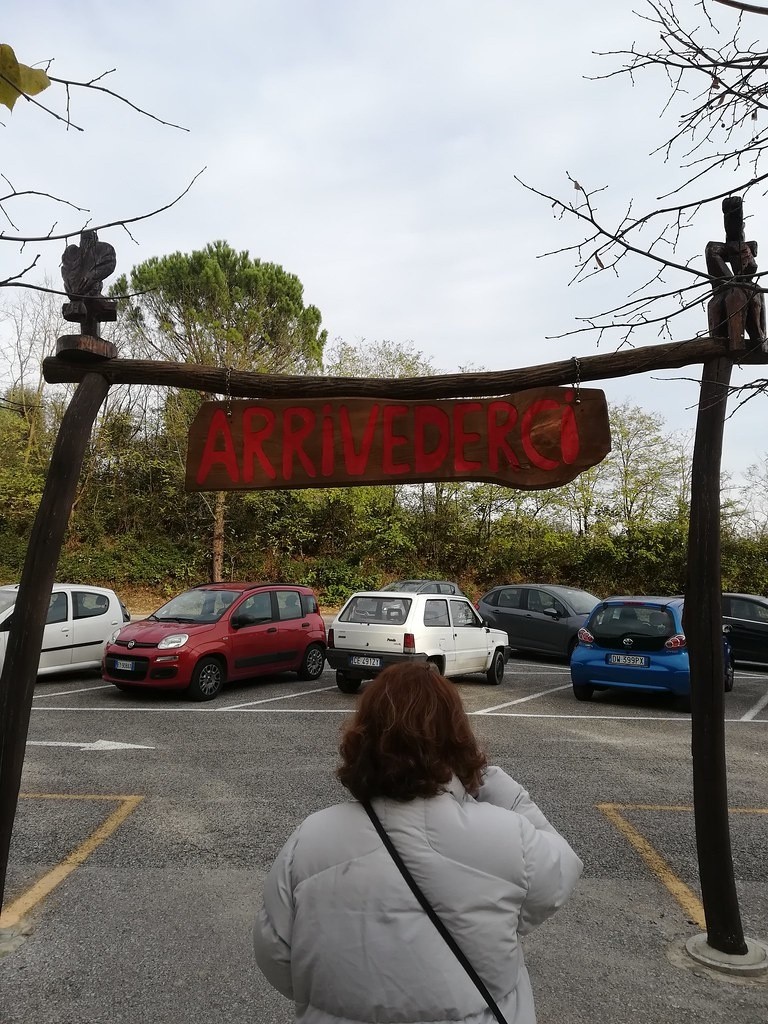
[388,608,402,623]
[498,594,520,608]
[91,596,108,614]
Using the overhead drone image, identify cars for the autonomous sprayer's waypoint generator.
[326,592,509,695]
[383,579,467,597]
[100,581,329,701]
[1,583,130,680]
[720,592,768,663]
[570,595,733,712]
[474,584,603,661]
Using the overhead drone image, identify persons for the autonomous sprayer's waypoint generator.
[253,661,585,1024]
